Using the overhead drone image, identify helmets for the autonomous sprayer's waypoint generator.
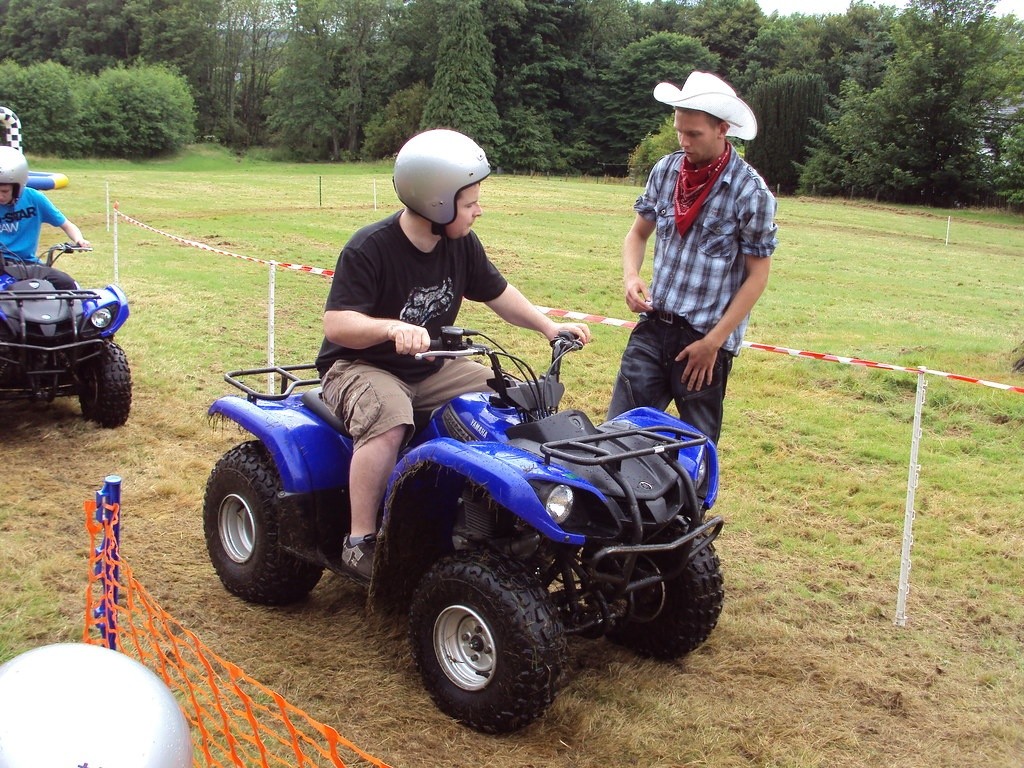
[393,129,490,224]
[0,145,28,197]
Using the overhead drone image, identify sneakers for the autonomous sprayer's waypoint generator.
[339,533,378,581]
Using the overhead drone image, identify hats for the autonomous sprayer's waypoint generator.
[653,71,758,140]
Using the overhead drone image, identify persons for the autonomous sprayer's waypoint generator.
[0,146,91,295]
[316,130,594,580]
[604,72,782,446]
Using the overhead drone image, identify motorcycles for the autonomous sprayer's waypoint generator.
[201,326,725,738]
[0,243,135,428]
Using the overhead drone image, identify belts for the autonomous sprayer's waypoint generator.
[646,308,690,328]
